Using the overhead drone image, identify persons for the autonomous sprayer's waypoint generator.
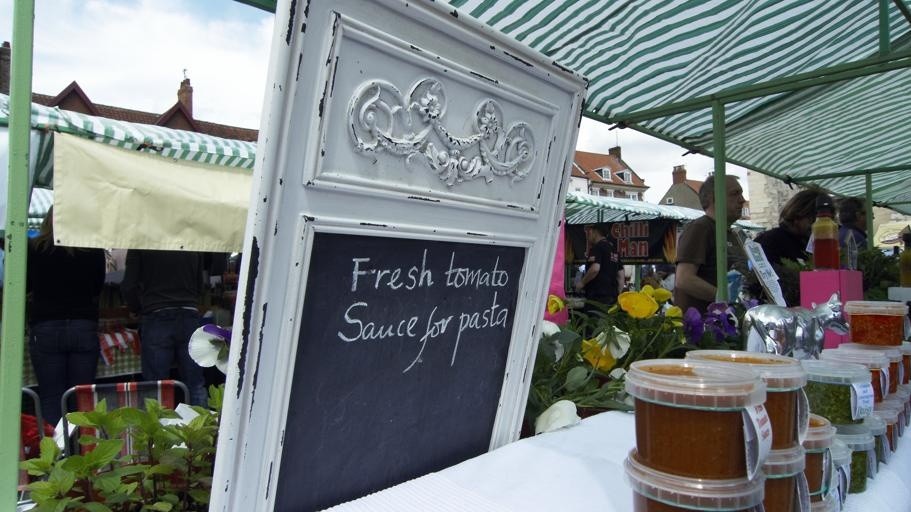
[27,205,106,427]
[573,224,625,341]
[674,175,875,336]
[122,250,209,411]
[899,234,911,287]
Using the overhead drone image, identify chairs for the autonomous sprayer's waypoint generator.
[61,381,192,473]
[21,385,47,480]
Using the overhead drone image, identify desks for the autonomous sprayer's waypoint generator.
[318,401,911,512]
[25,324,143,383]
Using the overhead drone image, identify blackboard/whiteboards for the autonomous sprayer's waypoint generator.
[253,211,538,512]
